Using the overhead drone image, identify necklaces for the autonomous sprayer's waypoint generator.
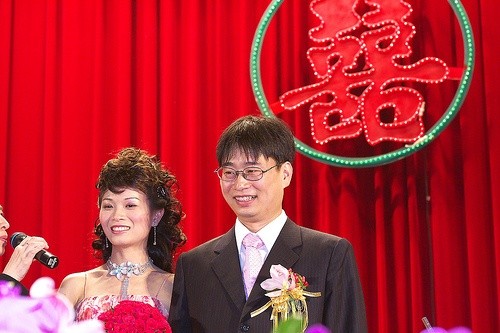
[105,257,156,281]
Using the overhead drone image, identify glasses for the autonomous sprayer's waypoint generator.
[213,162,285,181]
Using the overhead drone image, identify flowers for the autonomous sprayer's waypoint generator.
[251,264,321,333]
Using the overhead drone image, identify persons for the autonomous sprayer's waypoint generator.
[58,147,188,333]
[167,115,368,333]
[0,205,49,295]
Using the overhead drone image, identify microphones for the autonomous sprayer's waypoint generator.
[9,232,59,270]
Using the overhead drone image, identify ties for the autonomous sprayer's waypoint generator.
[242,233,264,301]
